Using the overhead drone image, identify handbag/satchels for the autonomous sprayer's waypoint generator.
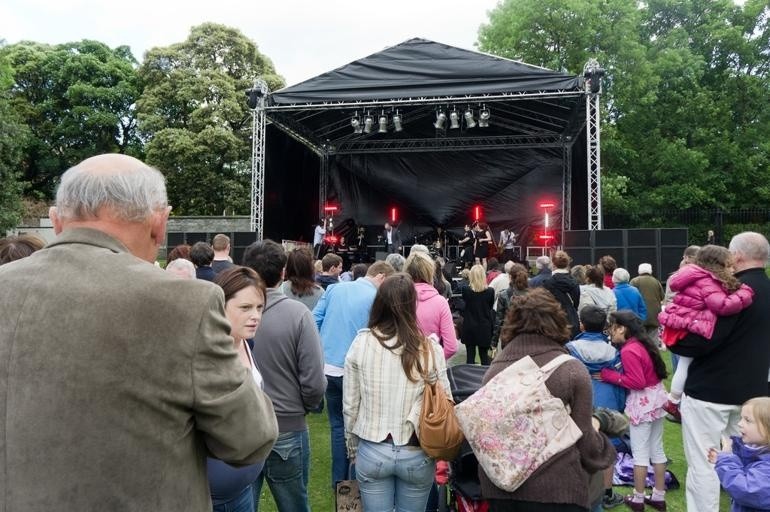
[337,480,362,512]
[419,338,465,461]
[453,354,584,492]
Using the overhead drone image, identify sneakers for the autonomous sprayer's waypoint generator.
[602,492,624,508]
[662,400,681,420]
[665,414,681,425]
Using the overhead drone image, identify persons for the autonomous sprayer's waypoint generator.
[0,152,279,512]
[165,216,769,511]
[1,215,48,265]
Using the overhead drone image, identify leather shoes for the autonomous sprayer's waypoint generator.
[644,495,666,512]
[624,496,645,512]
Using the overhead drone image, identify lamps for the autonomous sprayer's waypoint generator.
[346,103,492,133]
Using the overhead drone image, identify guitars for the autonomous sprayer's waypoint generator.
[499,233,519,254]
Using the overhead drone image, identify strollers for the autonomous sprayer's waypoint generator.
[439,364,491,512]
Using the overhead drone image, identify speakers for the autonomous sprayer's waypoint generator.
[563,229,593,269]
[660,228,688,285]
[594,229,626,270]
[626,228,659,282]
